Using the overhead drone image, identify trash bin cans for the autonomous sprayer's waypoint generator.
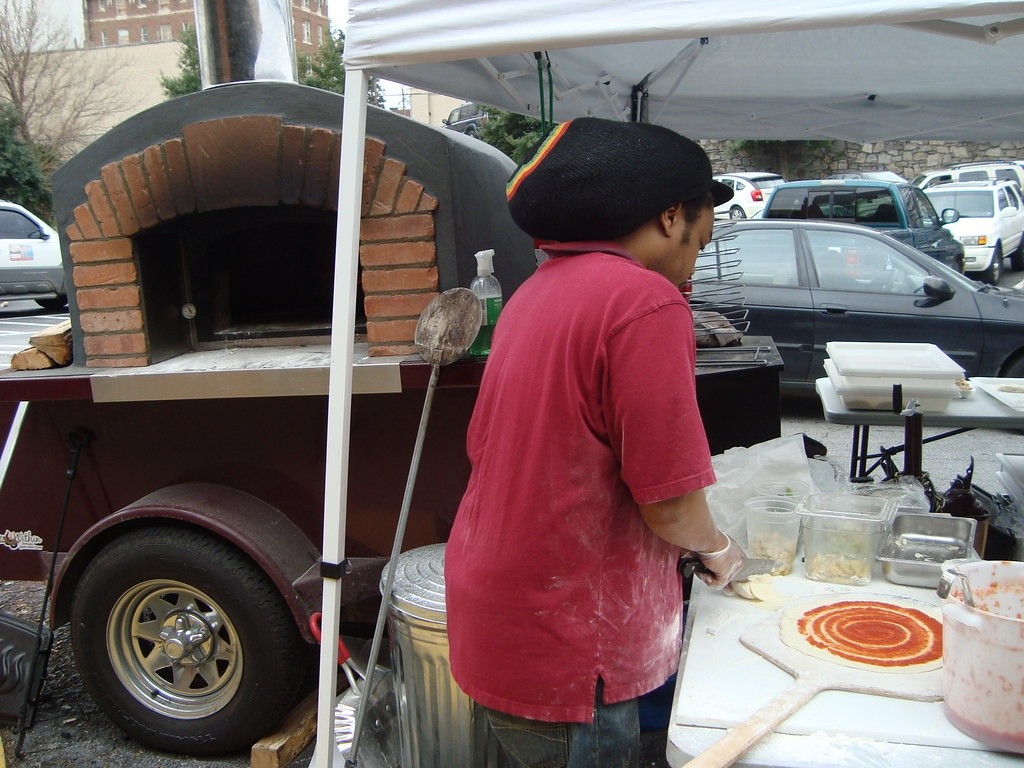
[380,542,476,768]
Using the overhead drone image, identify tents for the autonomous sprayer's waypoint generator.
[316,0,1024,768]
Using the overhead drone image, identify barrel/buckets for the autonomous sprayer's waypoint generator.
[936,556,1024,753]
[378,542,507,767]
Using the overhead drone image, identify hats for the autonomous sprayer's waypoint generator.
[506,115,733,242]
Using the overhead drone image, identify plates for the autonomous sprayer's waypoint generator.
[873,511,977,587]
[969,376,1023,413]
[957,388,973,397]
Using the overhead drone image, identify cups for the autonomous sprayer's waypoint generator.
[744,497,800,577]
[755,478,810,555]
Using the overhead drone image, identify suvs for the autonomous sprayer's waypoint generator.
[762,159,1024,286]
[439,104,498,141]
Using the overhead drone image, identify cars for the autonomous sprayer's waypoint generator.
[690,224,1024,397]
[713,171,787,219]
[0,198,69,308]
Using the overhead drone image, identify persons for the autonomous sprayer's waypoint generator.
[444,117,748,768]
[819,249,891,292]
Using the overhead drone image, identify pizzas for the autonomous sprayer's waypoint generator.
[779,596,944,674]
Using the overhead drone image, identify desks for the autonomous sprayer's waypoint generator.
[816,377,1024,477]
[665,513,1024,768]
